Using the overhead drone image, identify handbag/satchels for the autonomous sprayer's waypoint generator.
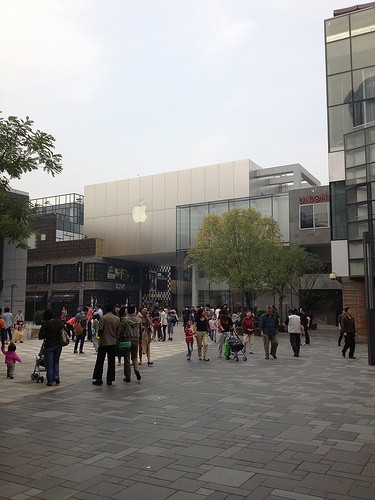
[59,320,70,347]
[118,340,132,350]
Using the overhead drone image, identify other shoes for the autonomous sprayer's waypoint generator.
[349,356,356,359]
[224,342,230,356]
[264,355,269,359]
[124,377,130,381]
[186,353,191,361]
[7,375,14,380]
[270,352,277,358]
[338,341,342,349]
[218,354,221,358]
[47,380,52,385]
[342,350,345,357]
[106,380,112,385]
[250,351,254,354]
[92,379,103,385]
[134,370,142,382]
[139,362,142,365]
[80,352,84,354]
[74,351,77,353]
[199,357,210,361]
[117,363,121,366]
[55,378,60,385]
[147,361,154,366]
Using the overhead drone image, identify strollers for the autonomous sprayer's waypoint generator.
[221,328,248,362]
[31,338,47,384]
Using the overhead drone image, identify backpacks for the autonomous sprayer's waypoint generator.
[74,317,83,336]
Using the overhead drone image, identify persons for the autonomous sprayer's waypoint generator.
[184,318,196,362]
[38,309,71,386]
[286,308,301,357]
[338,308,346,347]
[217,308,234,358]
[260,305,279,360]
[300,307,312,346]
[341,307,356,359]
[242,311,256,354]
[137,307,154,365]
[194,308,211,361]
[119,305,143,382]
[0,304,243,354]
[1,343,22,378]
[92,304,120,386]
